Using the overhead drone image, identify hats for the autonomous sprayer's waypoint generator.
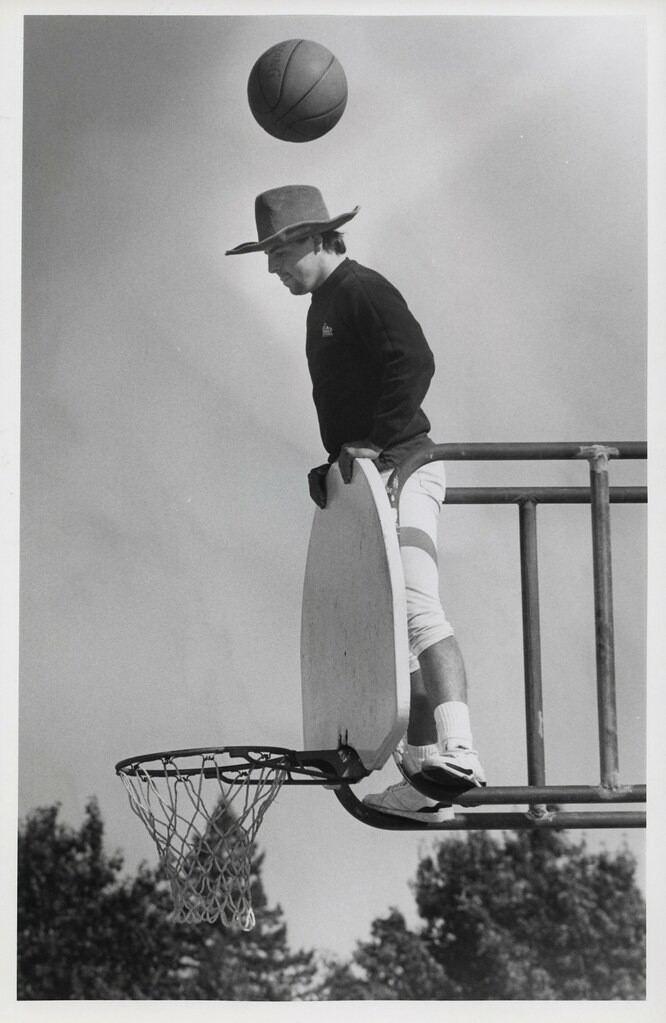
[225,185,362,256]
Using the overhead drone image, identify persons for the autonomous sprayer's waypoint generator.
[226,184,489,824]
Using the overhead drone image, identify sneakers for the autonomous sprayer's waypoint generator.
[362,778,455,821]
[422,748,488,788]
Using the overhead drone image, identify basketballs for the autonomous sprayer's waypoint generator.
[245,33,351,144]
